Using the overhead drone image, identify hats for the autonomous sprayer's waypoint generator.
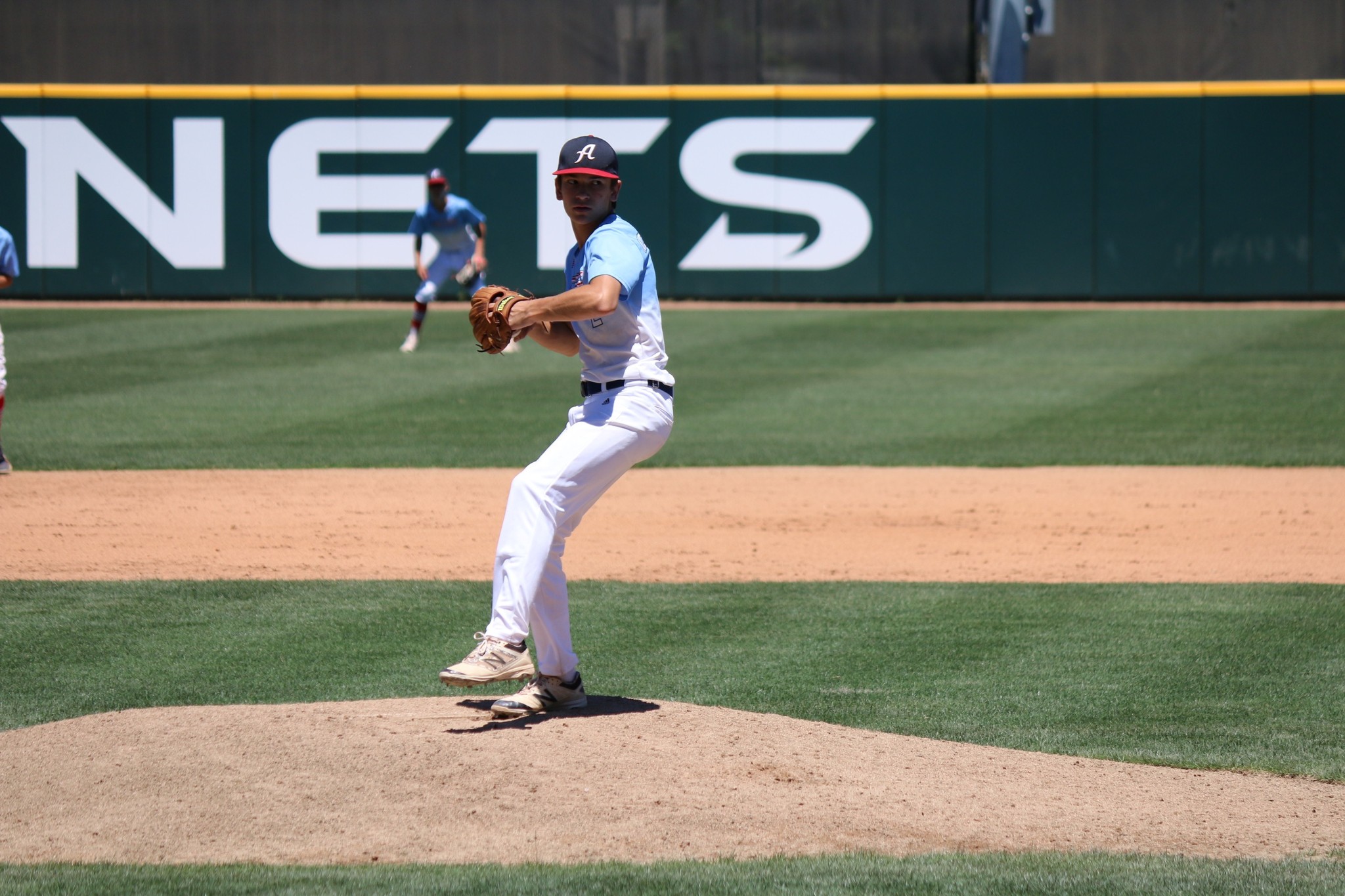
[553,134,621,182]
[426,167,446,184]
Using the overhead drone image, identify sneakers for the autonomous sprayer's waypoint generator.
[492,673,588,715]
[438,630,536,685]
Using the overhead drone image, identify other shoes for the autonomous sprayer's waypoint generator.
[399,333,420,353]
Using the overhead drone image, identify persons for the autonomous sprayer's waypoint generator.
[0,225,21,475]
[399,167,519,355]
[438,134,677,718]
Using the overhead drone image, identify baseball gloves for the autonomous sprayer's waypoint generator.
[468,285,533,356]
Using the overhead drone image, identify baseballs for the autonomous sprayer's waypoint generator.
[511,328,522,338]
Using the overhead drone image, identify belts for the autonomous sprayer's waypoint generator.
[582,381,673,397]
